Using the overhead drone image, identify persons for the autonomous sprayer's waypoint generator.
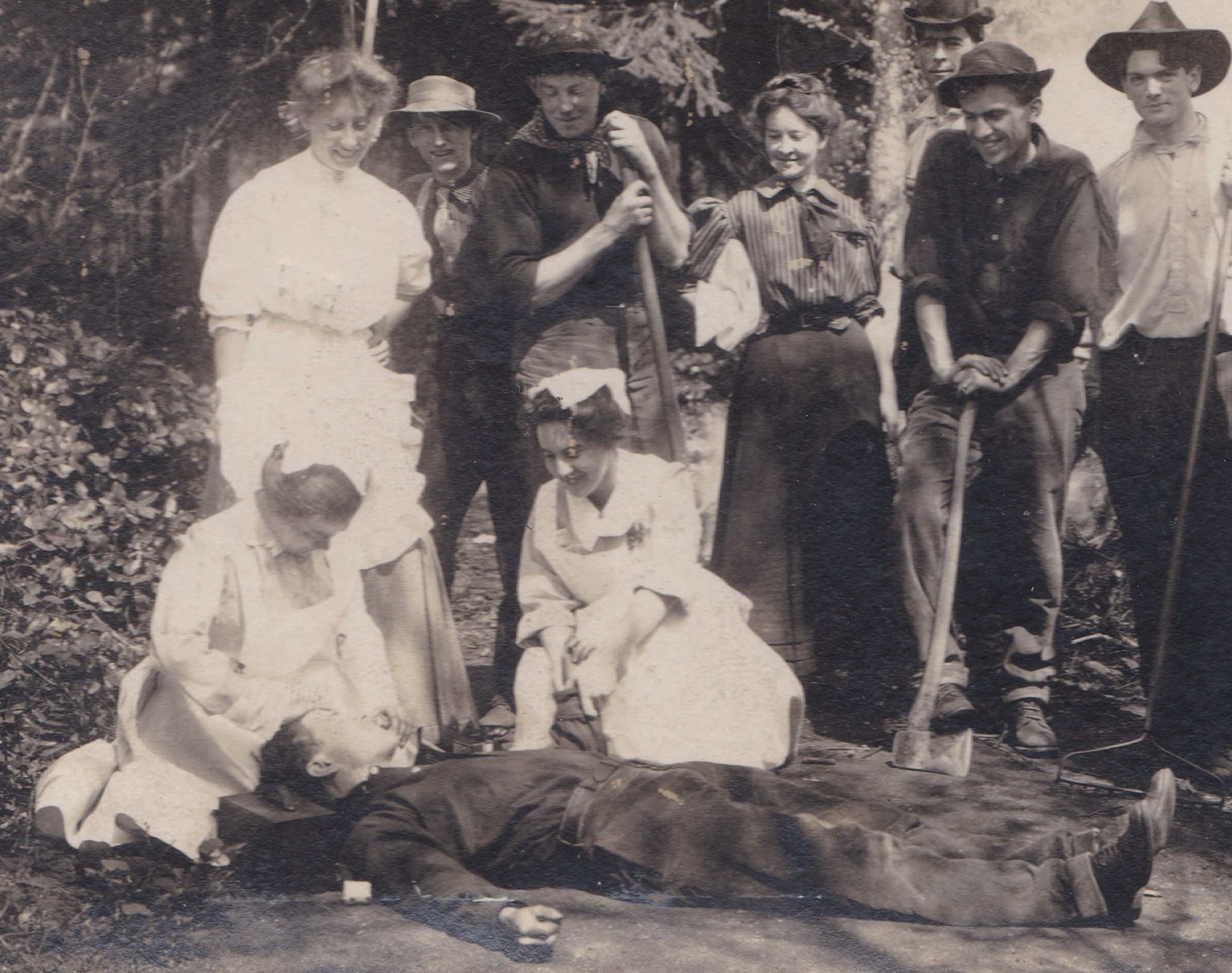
[888,0,997,281]
[386,73,533,728]
[684,69,905,722]
[258,657,1177,962]
[893,38,1109,753]
[485,16,697,582]
[511,363,800,772]
[200,49,474,761]
[1083,0,1232,789]
[33,438,412,867]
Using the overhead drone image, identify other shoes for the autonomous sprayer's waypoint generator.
[478,700,518,730]
[915,684,975,723]
[1098,760,1175,858]
[1010,697,1060,758]
[1092,801,1153,927]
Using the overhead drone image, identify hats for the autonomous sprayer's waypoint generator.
[902,1,995,27]
[384,78,504,137]
[503,21,634,75]
[1087,1,1232,96]
[935,40,1055,108]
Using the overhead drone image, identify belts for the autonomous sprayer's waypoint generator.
[562,755,629,874]
